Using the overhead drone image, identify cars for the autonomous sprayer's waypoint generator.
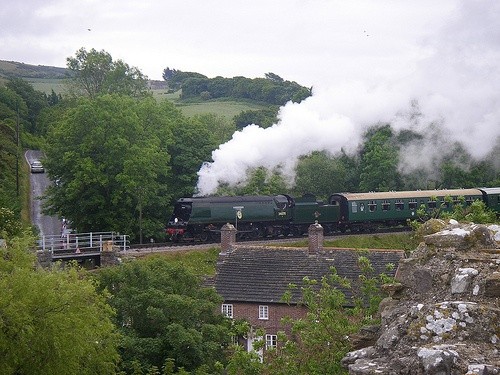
[30,160,44,173]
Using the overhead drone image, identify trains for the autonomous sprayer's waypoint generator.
[163,188,500,244]
